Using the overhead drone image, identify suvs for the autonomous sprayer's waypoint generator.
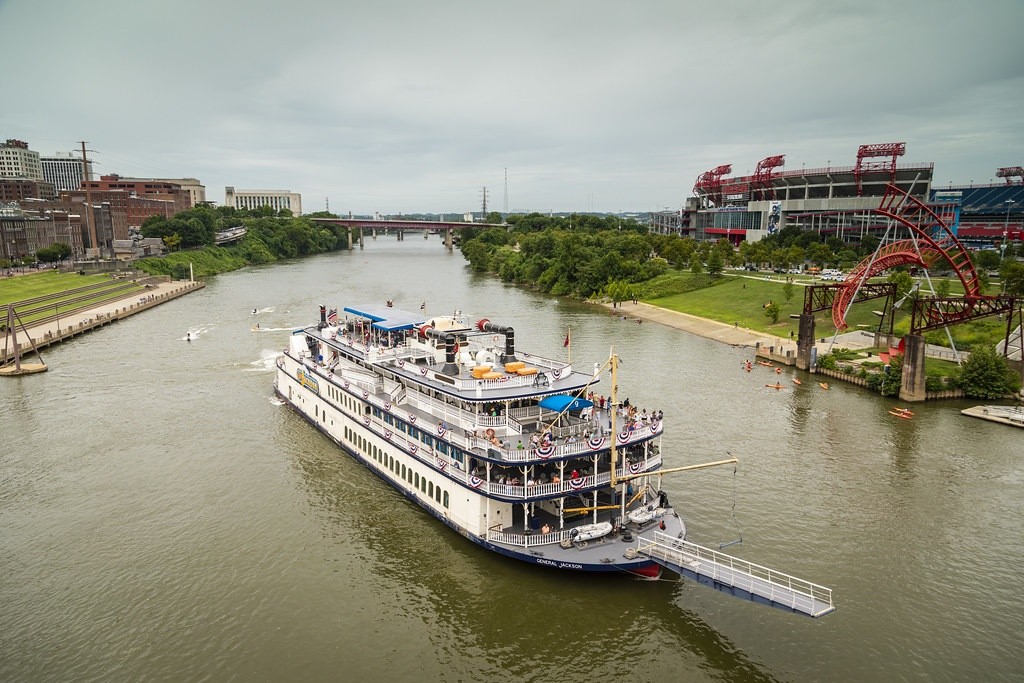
[745,266,759,272]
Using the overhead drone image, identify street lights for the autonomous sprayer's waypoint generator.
[1001,199,1015,259]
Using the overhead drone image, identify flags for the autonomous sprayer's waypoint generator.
[327,309,337,323]
[564,334,570,348]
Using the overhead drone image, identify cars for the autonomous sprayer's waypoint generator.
[833,276,846,282]
[734,266,745,271]
[774,269,787,274]
[789,269,802,274]
[30,262,47,269]
[821,275,832,280]
[808,265,820,270]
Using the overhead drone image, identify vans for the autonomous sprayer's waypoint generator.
[805,271,820,277]
[822,269,842,276]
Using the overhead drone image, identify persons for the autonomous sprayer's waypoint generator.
[745,346,827,387]
[788,330,794,339]
[614,299,637,308]
[766,274,817,286]
[140,294,154,303]
[391,374,440,400]
[309,337,337,374]
[49,330,52,338]
[743,284,745,288]
[188,333,190,338]
[255,309,256,312]
[735,321,738,328]
[438,389,663,500]
[257,324,259,328]
[762,300,772,309]
[996,313,1009,323]
[337,318,413,348]
[542,523,557,543]
[113,273,126,279]
[660,520,666,539]
[7,264,58,274]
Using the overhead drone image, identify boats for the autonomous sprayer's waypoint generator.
[895,407,915,415]
[793,378,801,384]
[775,367,781,372]
[745,364,752,371]
[819,382,828,389]
[765,384,786,388]
[272,303,836,618]
[888,411,911,418]
[758,361,774,366]
